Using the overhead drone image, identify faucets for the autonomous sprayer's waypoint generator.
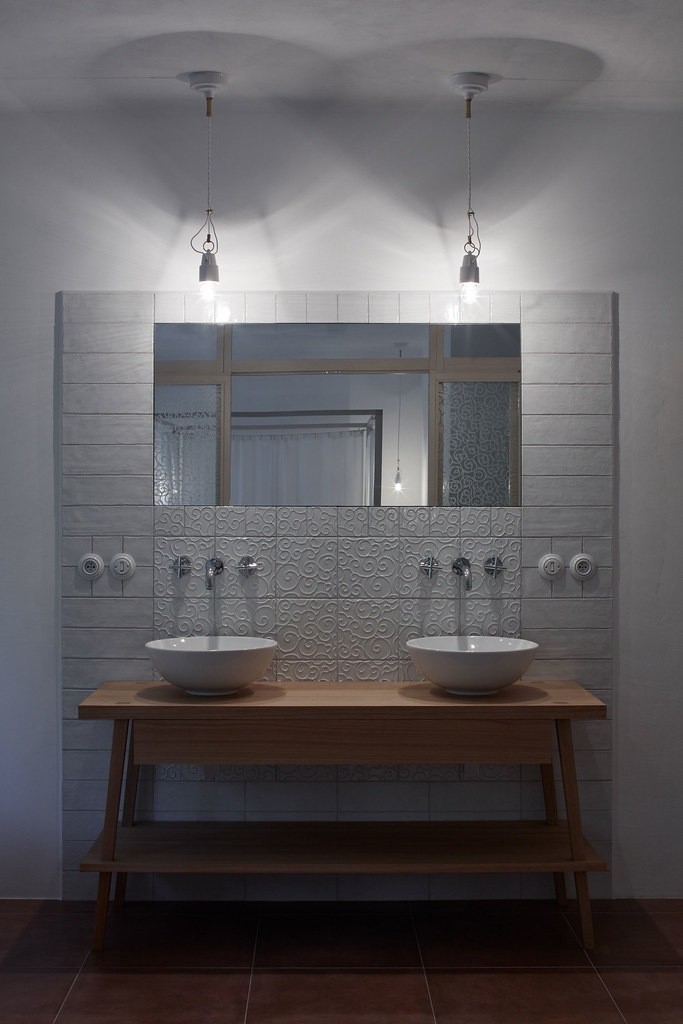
[204,557,224,591]
[452,557,473,591]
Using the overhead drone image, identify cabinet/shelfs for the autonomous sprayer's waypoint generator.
[76,679,609,949]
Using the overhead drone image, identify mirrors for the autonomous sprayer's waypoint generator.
[154,321,522,506]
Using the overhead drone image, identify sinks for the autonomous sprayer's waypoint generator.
[406,635,540,696]
[144,635,278,697]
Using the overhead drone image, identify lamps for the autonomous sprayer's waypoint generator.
[392,342,408,492]
[447,73,489,305]
[188,71,226,301]
